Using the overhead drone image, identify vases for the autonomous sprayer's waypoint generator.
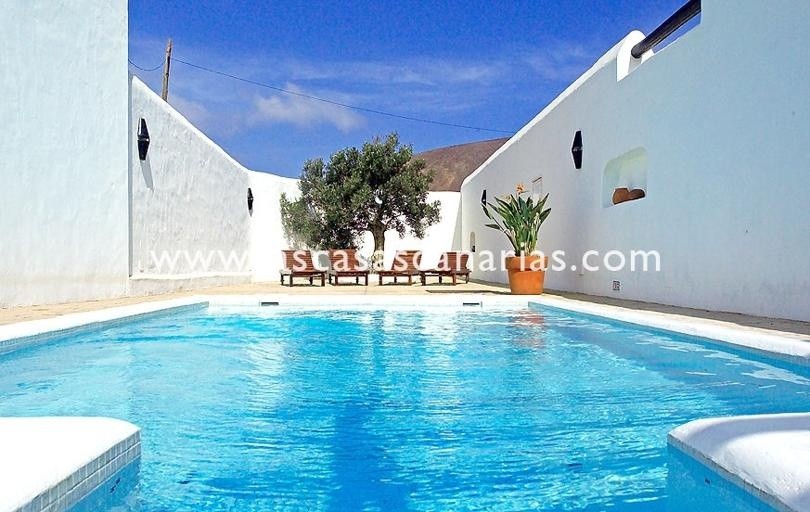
[505,255,549,295]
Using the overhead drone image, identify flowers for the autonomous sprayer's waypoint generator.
[480,182,551,257]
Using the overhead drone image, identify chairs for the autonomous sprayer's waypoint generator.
[328,248,371,286]
[424,251,474,284]
[280,247,327,287]
[377,249,424,287]
